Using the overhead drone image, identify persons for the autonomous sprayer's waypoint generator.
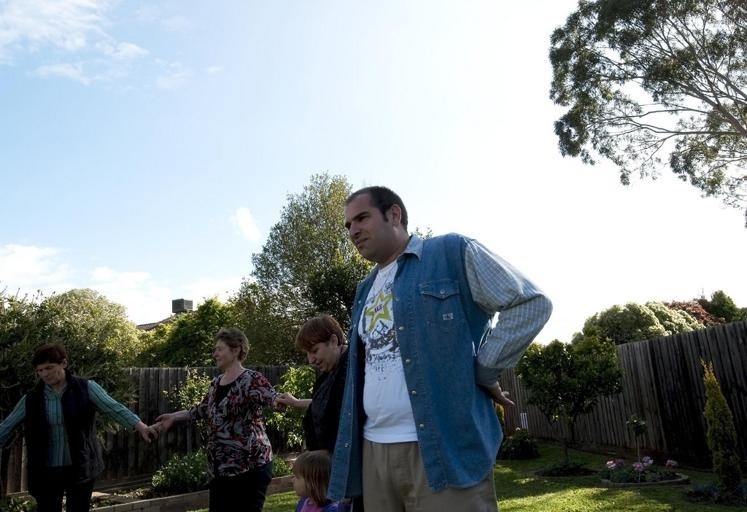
[327,187,552,512]
[0,342,157,512]
[149,327,286,512]
[292,450,351,512]
[277,314,350,512]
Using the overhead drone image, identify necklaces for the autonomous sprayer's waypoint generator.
[371,254,398,303]
